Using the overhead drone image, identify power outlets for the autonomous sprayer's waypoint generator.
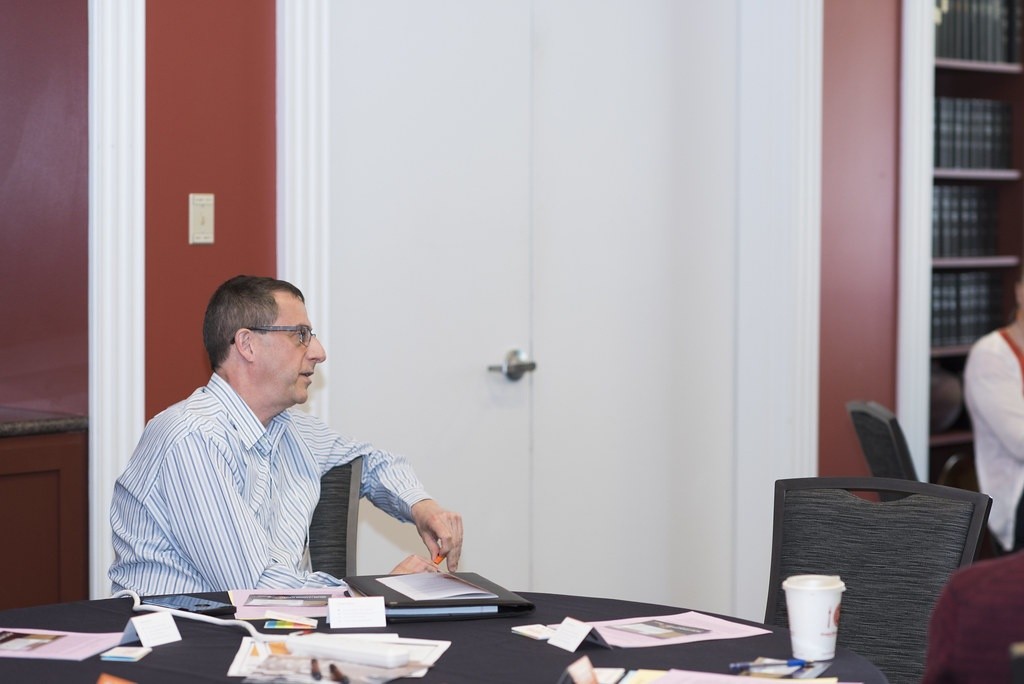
[188,193,215,245]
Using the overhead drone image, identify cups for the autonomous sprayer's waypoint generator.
[782,573,846,661]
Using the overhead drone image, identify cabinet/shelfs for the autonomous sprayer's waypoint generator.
[899,0,1024,502]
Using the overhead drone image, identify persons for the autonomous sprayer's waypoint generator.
[964,263,1024,557]
[107,275,464,598]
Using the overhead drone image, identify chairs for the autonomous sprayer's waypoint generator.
[310,455,367,580]
[763,476,992,684]
[850,398,974,502]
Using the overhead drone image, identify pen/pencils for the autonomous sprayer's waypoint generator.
[329,664,350,684]
[728,659,815,671]
[192,606,236,616]
[311,659,322,681]
[344,591,351,597]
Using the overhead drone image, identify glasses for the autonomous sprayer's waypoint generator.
[230,324,317,347]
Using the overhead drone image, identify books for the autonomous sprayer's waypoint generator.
[932,0,1023,434]
[344,572,536,622]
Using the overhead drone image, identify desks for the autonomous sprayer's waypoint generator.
[0,591,889,684]
